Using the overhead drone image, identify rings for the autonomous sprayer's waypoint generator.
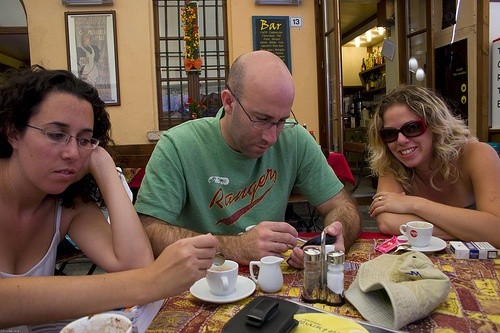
[378,197,382,201]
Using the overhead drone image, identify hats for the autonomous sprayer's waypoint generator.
[344,250,451,330]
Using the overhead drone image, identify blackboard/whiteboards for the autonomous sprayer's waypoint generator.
[251,15,292,75]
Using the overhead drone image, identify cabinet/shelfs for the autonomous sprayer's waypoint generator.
[358,62,386,112]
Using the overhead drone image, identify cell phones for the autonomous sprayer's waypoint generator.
[287,233,336,266]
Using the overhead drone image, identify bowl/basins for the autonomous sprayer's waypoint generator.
[58,313,133,333]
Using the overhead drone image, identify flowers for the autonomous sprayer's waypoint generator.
[180,3,200,62]
[184,97,208,118]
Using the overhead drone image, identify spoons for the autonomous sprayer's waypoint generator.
[213,250,225,266]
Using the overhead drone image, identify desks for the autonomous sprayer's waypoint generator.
[146,230,500,333]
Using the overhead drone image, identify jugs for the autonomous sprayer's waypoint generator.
[249,255,284,294]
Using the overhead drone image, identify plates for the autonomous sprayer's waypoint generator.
[396,234,447,252]
[189,275,257,303]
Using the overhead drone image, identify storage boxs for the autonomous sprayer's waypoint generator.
[449,241,498,259]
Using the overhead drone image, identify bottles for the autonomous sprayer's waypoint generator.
[325,250,345,303]
[364,73,386,91]
[303,246,322,301]
[361,46,385,72]
[344,90,369,128]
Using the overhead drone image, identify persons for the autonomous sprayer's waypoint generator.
[135,50,361,268]
[0,65,218,333]
[364,85,500,250]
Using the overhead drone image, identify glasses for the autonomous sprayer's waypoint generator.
[224,85,298,131]
[25,124,100,150]
[378,119,427,144]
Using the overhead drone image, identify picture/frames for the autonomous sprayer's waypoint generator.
[64,10,121,106]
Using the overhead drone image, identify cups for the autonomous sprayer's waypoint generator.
[206,259,239,296]
[399,221,434,247]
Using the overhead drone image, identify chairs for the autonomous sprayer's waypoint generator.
[339,142,365,196]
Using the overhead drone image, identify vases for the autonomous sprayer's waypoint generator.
[192,112,198,120]
